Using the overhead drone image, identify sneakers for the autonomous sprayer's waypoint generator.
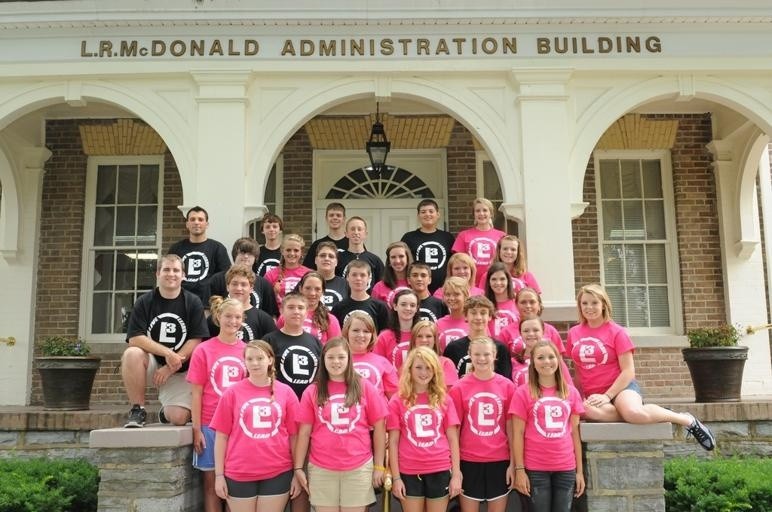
[124,404,146,428]
[158,406,169,423]
[685,412,716,450]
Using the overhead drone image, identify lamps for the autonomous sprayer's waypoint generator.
[363,98,393,178]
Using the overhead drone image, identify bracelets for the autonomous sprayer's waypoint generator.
[393,477,401,480]
[375,465,384,471]
[514,465,526,470]
[215,472,224,476]
[603,391,612,400]
[294,338,389,512]
[293,468,303,470]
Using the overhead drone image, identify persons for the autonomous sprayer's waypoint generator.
[183,296,257,512]
[507,337,584,511]
[121,254,210,429]
[446,335,516,512]
[172,197,542,345]
[443,295,514,382]
[215,339,303,512]
[385,345,462,512]
[409,321,458,395]
[564,284,715,452]
[500,288,565,353]
[511,316,574,386]
[341,310,398,400]
[261,293,324,401]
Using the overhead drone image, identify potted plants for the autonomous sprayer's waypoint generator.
[678,320,751,405]
[34,336,103,410]
